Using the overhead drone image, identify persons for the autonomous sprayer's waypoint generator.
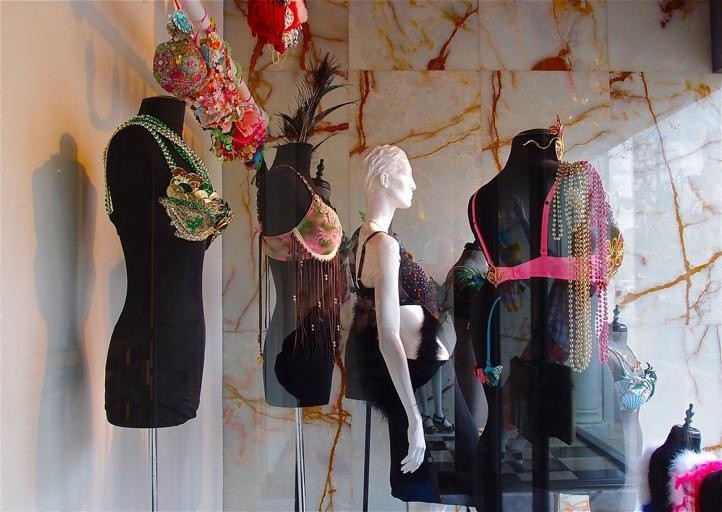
[258,142,348,408]
[468,125,624,512]
[442,238,511,512]
[341,144,452,476]
[648,402,722,511]
[103,97,237,428]
[589,304,656,512]
